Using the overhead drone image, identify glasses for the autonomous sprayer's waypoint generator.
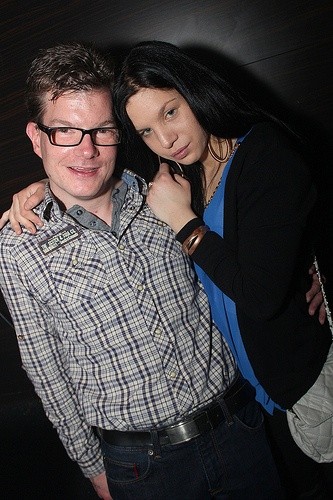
[38,123,123,146]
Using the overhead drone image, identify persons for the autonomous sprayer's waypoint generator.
[0,40,327,500]
[2,44,333,500]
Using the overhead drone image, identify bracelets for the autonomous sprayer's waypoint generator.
[182,225,210,255]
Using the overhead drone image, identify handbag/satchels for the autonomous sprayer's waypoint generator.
[287,256,333,464]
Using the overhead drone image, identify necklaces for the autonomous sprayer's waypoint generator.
[204,143,240,208]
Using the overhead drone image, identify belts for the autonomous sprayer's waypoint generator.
[90,369,256,449]
